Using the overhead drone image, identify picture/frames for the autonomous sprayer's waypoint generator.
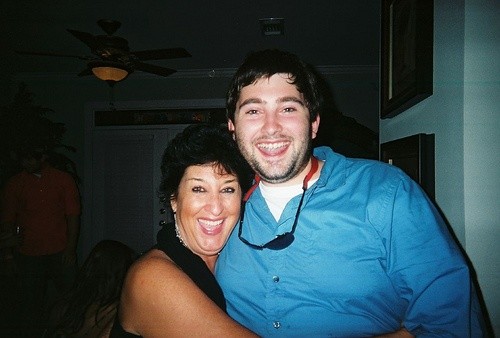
[379,133,435,202]
[381,0,434,119]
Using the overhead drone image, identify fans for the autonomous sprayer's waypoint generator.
[66,28,192,77]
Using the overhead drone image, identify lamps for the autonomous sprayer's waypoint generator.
[91,19,129,109]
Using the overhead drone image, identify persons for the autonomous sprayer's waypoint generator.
[0,138,81,305]
[212,48,495,338]
[108,120,263,338]
[43,239,137,338]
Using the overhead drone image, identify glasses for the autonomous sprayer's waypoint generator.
[238,187,306,250]
